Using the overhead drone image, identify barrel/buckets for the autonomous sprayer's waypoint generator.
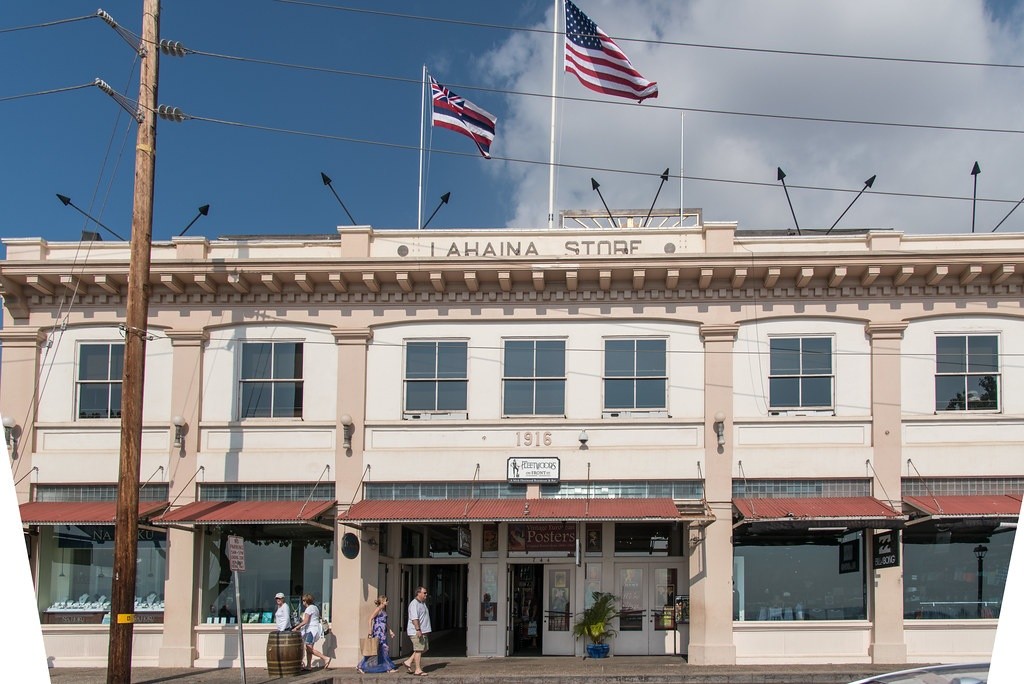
[266,631,304,678]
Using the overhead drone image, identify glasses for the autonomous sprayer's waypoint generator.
[420,592,427,595]
[382,598,388,602]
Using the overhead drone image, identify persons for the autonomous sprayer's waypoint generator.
[356,595,398,674]
[403,587,432,675]
[292,594,331,671]
[274,593,292,632]
[528,600,537,648]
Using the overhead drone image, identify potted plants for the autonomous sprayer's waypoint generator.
[570,591,625,657]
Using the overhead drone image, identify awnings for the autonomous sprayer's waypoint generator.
[901,494,1024,526]
[149,500,338,532]
[335,497,717,529]
[731,496,908,531]
[18,502,170,534]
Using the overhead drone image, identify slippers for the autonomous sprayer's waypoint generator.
[413,671,429,677]
[323,657,331,669]
[402,661,413,674]
[301,666,311,671]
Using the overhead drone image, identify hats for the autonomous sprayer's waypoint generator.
[274,593,286,599]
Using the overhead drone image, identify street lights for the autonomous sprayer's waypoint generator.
[973,545,989,619]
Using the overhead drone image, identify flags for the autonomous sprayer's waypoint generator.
[428,75,497,159]
[562,0,658,102]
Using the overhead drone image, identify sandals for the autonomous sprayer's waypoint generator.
[386,669,399,674]
[356,666,365,675]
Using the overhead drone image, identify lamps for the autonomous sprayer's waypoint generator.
[2,414,18,449]
[714,410,727,446]
[340,414,353,448]
[172,415,186,447]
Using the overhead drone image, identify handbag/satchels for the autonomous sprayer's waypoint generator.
[318,615,329,635]
[363,634,379,656]
